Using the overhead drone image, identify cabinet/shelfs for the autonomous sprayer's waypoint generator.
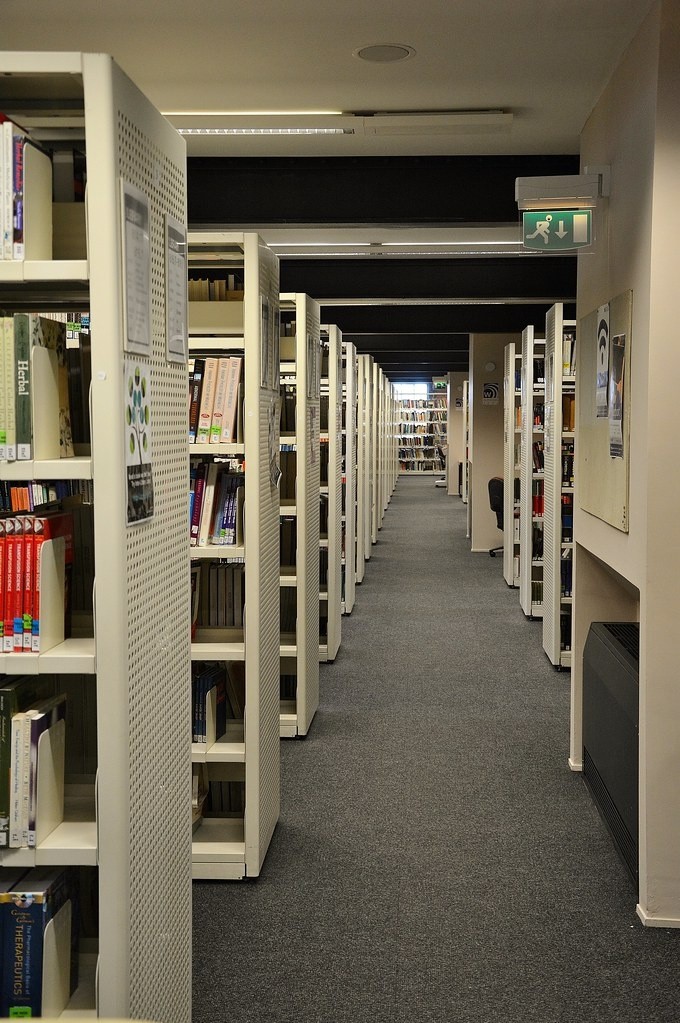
[0,49,580,1023]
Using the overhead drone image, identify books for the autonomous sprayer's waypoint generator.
[1,112,577,1017]
